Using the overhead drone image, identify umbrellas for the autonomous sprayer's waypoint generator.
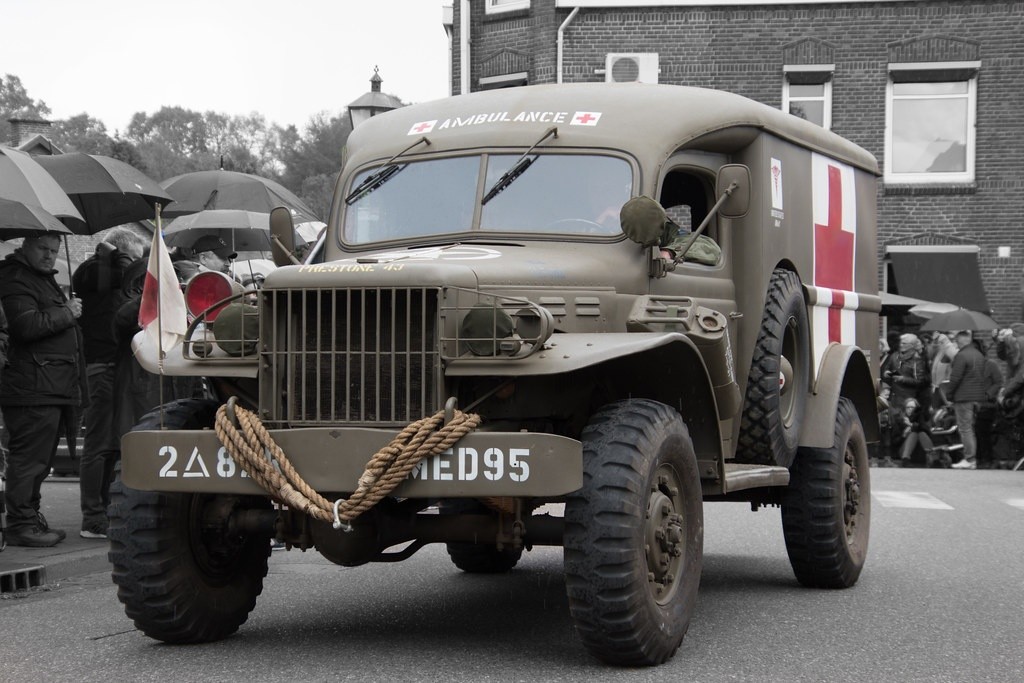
[161,207,309,282]
[293,221,327,246]
[158,153,322,222]
[909,303,964,319]
[918,309,1001,333]
[0,147,86,223]
[31,155,176,299]
[0,198,75,235]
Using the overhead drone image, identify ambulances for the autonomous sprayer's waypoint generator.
[106,84,887,669]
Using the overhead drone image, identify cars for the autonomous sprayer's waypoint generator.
[0,242,86,475]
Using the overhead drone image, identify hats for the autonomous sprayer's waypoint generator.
[191,235,238,259]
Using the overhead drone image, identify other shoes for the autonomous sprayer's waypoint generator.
[38,520,66,541]
[951,459,977,469]
[5,527,60,547]
[79,525,108,538]
[902,458,910,468]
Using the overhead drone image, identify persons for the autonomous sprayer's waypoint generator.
[0,229,83,546]
[133,233,264,424]
[70,227,146,539]
[868,322,1023,469]
[583,187,722,267]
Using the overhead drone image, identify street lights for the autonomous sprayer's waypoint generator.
[347,64,406,131]
